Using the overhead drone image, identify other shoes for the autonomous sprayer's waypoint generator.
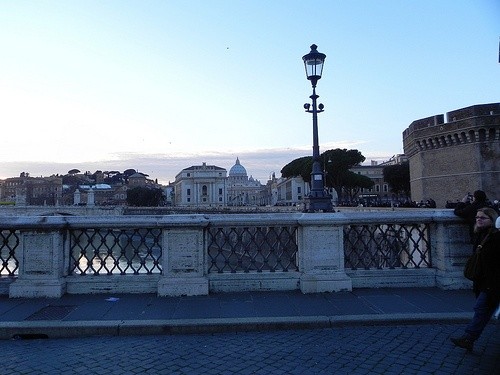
[451,336,473,352]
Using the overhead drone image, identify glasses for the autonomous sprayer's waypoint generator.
[475,216,489,220]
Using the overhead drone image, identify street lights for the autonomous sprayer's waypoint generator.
[301,43,336,213]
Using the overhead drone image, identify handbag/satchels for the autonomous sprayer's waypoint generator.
[464,249,482,281]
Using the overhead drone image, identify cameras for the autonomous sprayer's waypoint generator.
[467,195,473,202]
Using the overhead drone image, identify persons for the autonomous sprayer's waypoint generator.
[337,189,500,351]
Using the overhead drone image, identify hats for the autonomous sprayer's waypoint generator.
[478,208,498,222]
